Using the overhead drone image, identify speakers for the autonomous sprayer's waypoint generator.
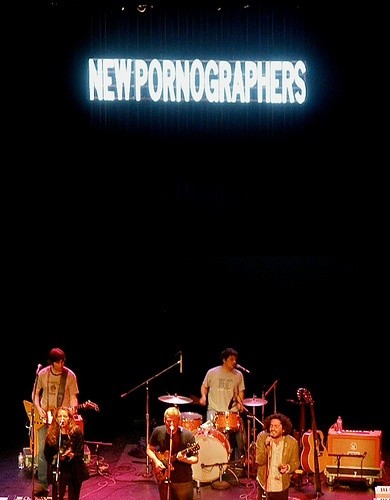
[330,435,381,468]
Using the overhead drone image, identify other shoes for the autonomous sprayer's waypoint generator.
[34,483,49,497]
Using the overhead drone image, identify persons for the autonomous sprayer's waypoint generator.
[199,347,247,455]
[146,406,199,500]
[43,405,92,500]
[31,348,79,495]
[253,413,301,500]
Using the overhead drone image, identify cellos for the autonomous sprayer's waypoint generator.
[297,388,328,497]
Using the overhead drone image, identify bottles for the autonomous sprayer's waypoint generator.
[337,416,342,431]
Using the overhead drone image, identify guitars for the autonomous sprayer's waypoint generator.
[46,447,72,486]
[153,443,200,485]
[24,400,99,431]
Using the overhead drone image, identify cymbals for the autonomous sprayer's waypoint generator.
[242,398,268,406]
[158,396,192,404]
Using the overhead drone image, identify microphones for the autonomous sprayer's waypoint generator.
[266,433,273,445]
[170,426,174,439]
[59,420,64,426]
[36,364,42,374]
[236,363,250,373]
[179,351,183,373]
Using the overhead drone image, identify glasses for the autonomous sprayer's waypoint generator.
[270,424,281,428]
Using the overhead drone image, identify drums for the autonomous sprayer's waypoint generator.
[180,412,202,432]
[192,428,231,482]
[215,411,240,432]
